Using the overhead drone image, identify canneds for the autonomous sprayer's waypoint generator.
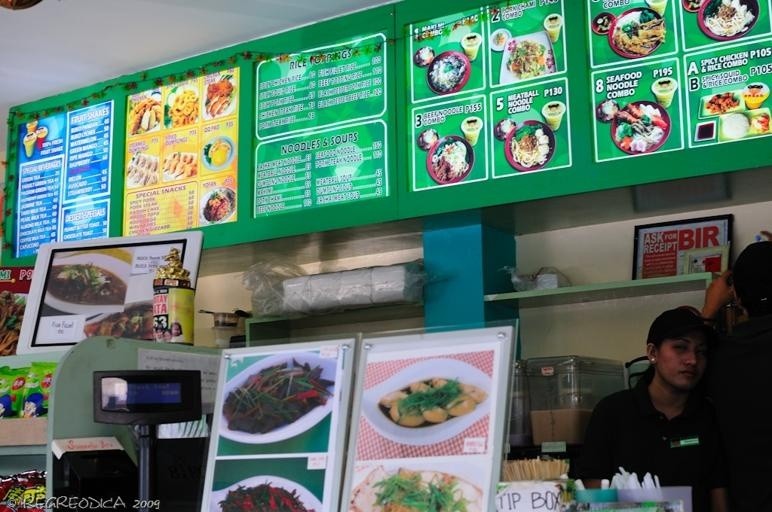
[153,285,196,346]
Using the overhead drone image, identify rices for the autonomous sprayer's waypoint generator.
[722,114,749,138]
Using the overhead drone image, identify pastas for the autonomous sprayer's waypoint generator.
[510,137,549,168]
[432,144,469,177]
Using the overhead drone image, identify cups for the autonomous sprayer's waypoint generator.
[742,81,770,110]
[460,116,483,147]
[543,13,563,44]
[36,126,49,151]
[651,77,679,110]
[461,32,482,63]
[645,0,668,18]
[541,100,566,133]
[26,120,38,133]
[23,132,37,159]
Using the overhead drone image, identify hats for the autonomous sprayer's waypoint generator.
[648,310,714,341]
[733,241,772,305]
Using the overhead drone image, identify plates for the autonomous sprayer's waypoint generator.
[591,12,616,36]
[211,474,322,512]
[596,99,620,122]
[499,31,558,85]
[504,119,556,172]
[494,119,516,141]
[490,28,512,52]
[694,120,716,143]
[682,0,706,14]
[608,7,666,60]
[426,135,475,185]
[219,351,338,445]
[199,186,238,226]
[360,356,493,448]
[414,47,435,67]
[426,50,471,95]
[698,0,760,42]
[201,135,235,173]
[610,100,671,156]
[43,252,132,315]
[417,128,439,151]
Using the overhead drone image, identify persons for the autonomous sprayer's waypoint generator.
[155,322,184,343]
[700,230,772,511]
[572,305,731,512]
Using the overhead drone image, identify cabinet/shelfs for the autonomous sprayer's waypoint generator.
[0,336,222,512]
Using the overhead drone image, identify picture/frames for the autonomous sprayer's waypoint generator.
[632,213,734,279]
[682,245,730,275]
